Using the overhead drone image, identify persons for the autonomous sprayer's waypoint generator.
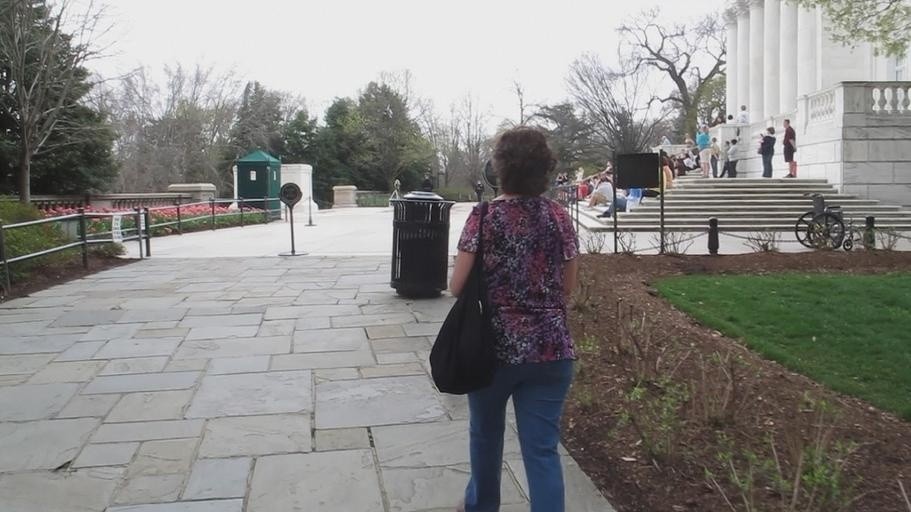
[782,119,798,177]
[475,180,485,202]
[758,126,777,177]
[450,126,581,509]
[557,106,749,218]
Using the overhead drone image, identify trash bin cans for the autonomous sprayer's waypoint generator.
[388,191,456,299]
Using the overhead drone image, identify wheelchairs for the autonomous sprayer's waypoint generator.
[797,187,868,255]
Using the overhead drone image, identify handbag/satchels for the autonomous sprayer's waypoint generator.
[758,146,769,155]
[430,301,499,395]
